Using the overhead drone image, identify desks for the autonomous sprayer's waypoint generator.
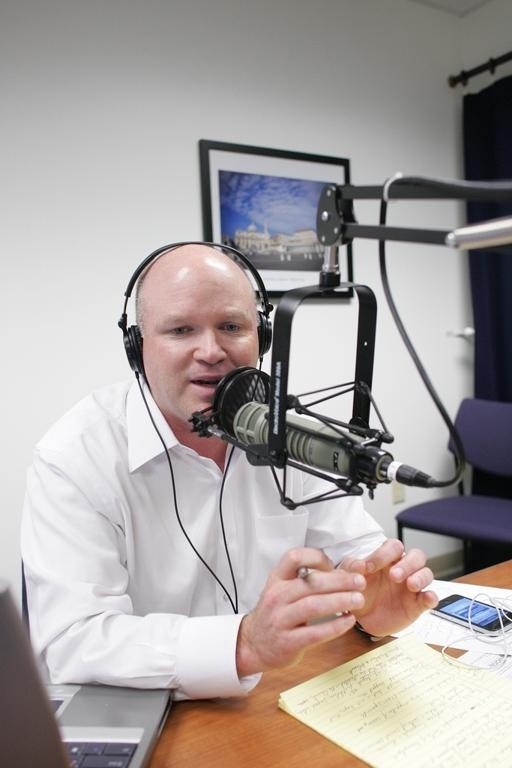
[144,558,512,768]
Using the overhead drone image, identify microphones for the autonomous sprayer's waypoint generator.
[227,401,440,490]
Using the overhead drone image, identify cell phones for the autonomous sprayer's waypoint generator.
[429,592,510,638]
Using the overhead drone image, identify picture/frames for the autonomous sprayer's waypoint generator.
[198,138,355,298]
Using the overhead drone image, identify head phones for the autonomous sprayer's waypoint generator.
[118,239,275,373]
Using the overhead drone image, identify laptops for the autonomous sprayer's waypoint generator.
[2,588,176,768]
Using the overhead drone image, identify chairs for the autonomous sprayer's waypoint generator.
[394,396,511,569]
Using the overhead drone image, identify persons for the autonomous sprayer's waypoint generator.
[15,242,440,704]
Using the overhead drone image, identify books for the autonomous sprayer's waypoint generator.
[273,634,512,768]
